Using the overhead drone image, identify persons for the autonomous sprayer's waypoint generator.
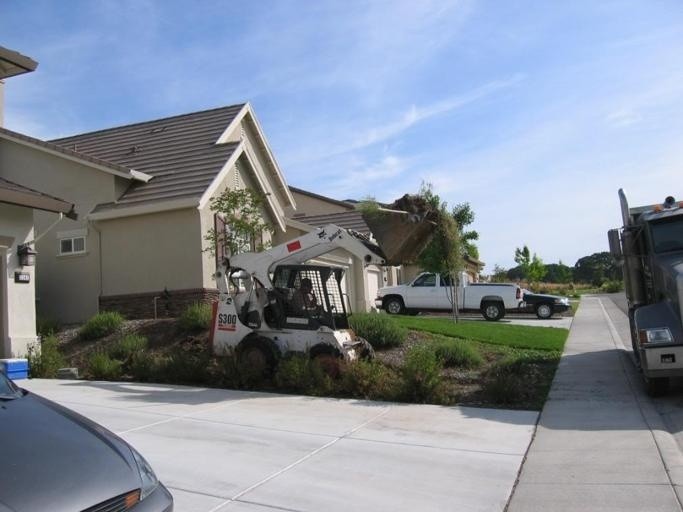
[288,278,336,331]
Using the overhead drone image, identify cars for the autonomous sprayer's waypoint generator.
[504,288,572,319]
[0,369,174,512]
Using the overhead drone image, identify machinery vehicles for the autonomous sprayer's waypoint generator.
[208,208,439,380]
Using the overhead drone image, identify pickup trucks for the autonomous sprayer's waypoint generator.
[377,271,527,321]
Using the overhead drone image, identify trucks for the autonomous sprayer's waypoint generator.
[608,188,683,396]
